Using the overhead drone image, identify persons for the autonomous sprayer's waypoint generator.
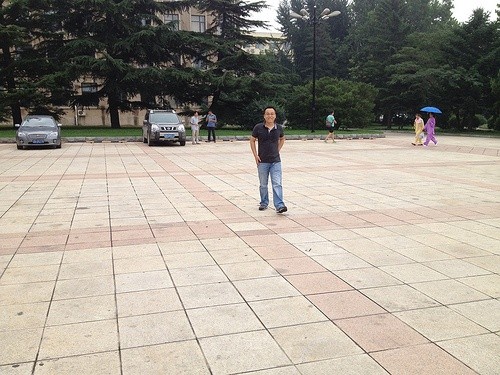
[190,111,206,144]
[250,107,288,213]
[412,113,424,146]
[205,109,217,143]
[423,113,438,146]
[324,111,337,143]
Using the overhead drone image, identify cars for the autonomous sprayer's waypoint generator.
[15,114,63,150]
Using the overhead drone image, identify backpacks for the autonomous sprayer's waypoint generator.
[326,120,331,128]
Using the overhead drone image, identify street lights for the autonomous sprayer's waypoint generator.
[289,4,341,132]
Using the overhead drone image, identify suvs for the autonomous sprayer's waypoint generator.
[142,109,186,146]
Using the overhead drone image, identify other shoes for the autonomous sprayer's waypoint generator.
[259,205,267,210]
[324,140,336,143]
[276,207,287,213]
[412,141,439,147]
[192,140,216,144]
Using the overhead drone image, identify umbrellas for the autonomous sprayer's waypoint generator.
[420,106,442,113]
